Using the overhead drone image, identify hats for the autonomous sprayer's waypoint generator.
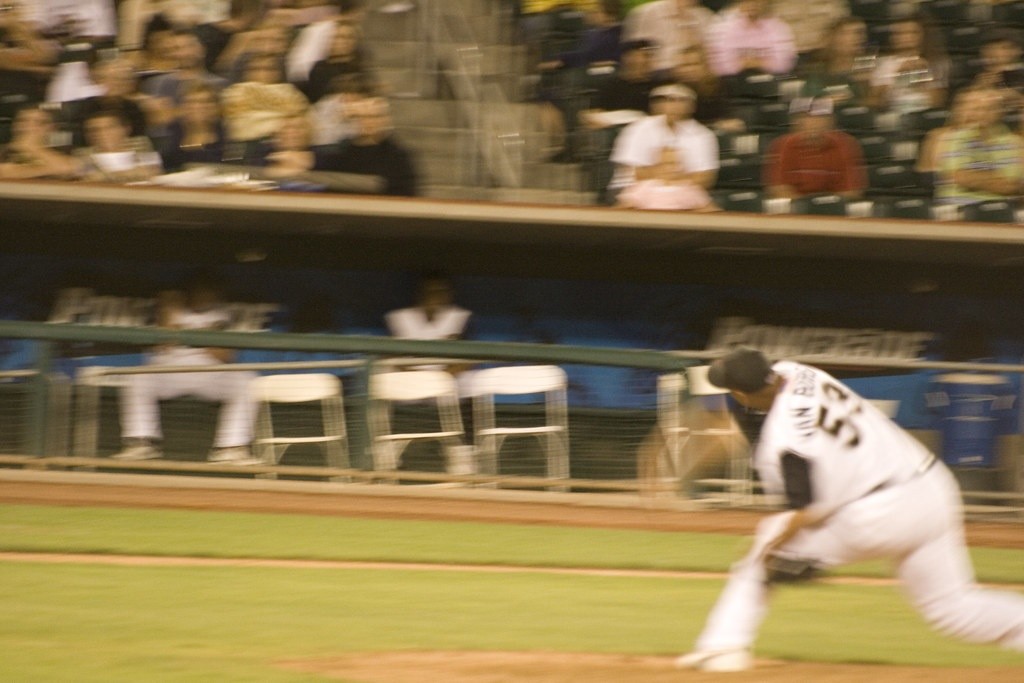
[651,81,698,100]
[707,347,775,393]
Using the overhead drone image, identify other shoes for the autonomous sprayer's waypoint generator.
[207,448,268,466]
[108,445,166,459]
[676,647,755,673]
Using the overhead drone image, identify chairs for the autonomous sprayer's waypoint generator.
[251,364,761,504]
[543,23,1024,222]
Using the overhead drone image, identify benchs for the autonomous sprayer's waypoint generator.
[73,365,764,479]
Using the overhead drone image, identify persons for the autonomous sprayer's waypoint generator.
[515,0,1024,224]
[0,0,413,197]
[385,276,469,368]
[675,346,1023,671]
[113,278,264,466]
